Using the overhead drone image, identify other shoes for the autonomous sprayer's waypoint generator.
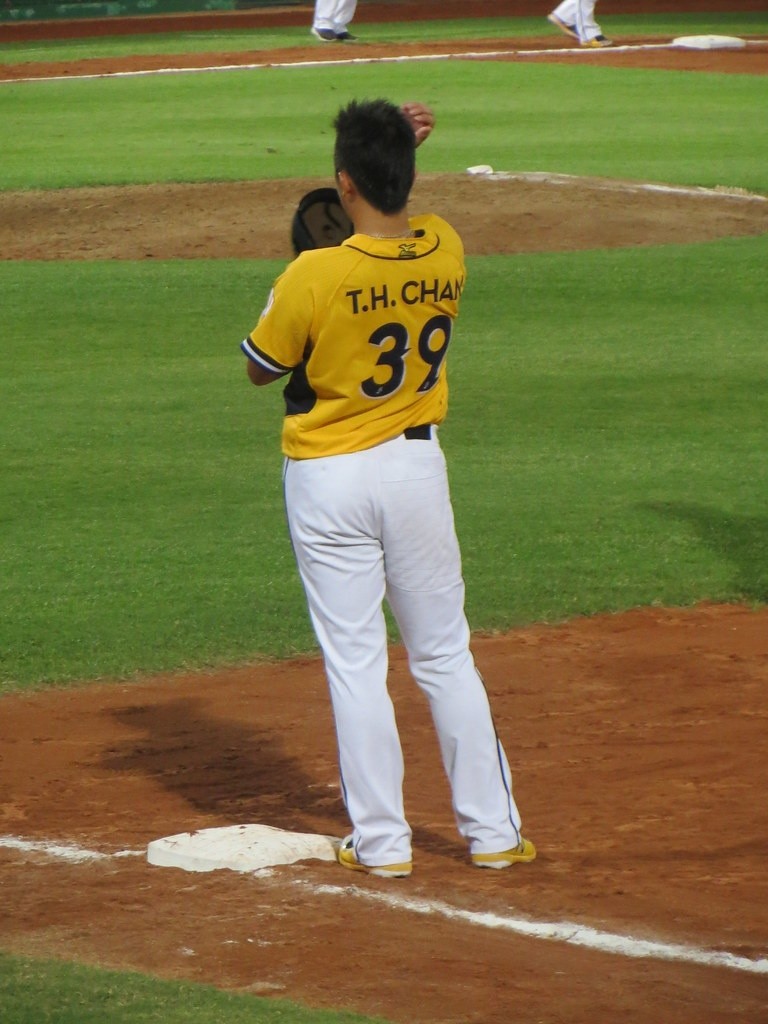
[312,26,337,41]
[337,31,359,42]
[548,12,580,38]
[580,35,613,47]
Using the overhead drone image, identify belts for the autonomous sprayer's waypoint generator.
[403,424,431,440]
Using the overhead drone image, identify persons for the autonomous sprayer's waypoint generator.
[547,0,613,48]
[240,97,537,878]
[310,0,361,42]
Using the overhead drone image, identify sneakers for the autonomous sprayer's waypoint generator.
[470,836,537,869]
[338,834,413,878]
